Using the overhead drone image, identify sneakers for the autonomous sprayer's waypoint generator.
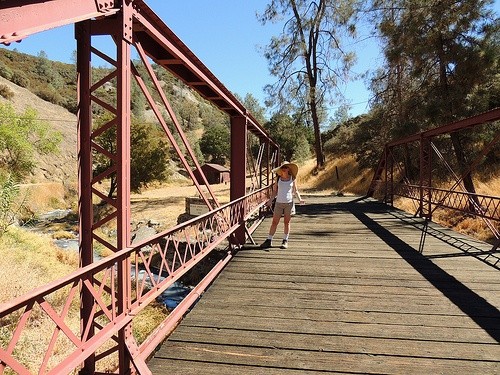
[280,240,288,249]
[260,239,271,247]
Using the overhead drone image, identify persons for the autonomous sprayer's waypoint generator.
[260,161,307,250]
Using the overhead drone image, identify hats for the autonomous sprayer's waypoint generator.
[271,161,298,179]
